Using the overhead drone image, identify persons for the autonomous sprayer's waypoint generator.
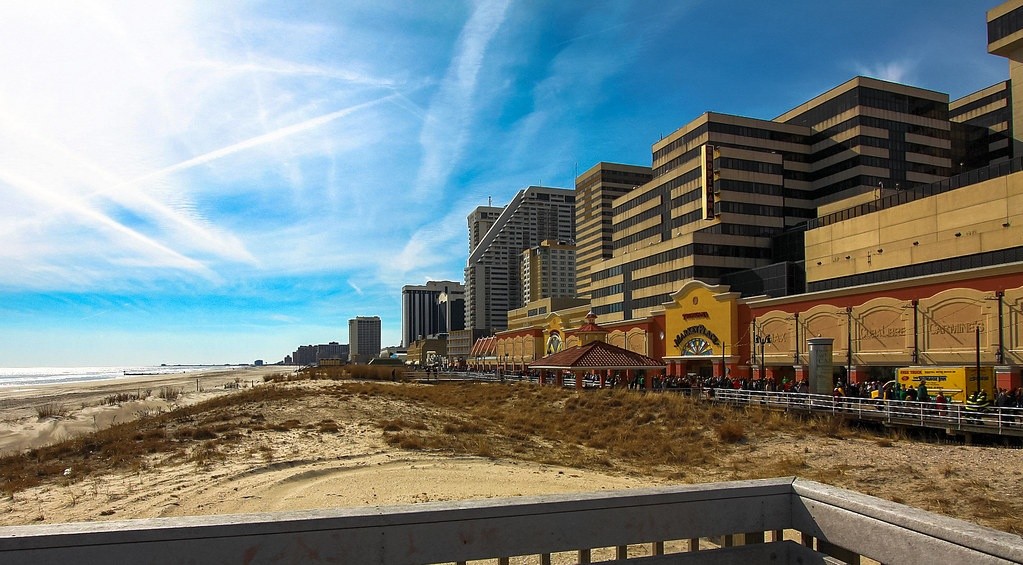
[639,377,644,389]
[585,372,600,381]
[483,368,495,374]
[391,369,396,381]
[433,367,437,379]
[607,375,619,386]
[630,378,635,389]
[833,377,884,411]
[563,373,576,379]
[651,374,809,408]
[965,388,1023,426]
[895,380,948,415]
[426,367,430,377]
[517,369,553,378]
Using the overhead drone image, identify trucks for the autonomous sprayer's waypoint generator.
[875,364,993,407]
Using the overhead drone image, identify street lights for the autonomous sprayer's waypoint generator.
[754,334,771,391]
